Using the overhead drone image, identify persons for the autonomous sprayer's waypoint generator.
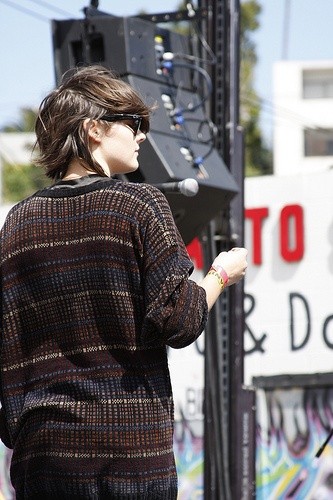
[0,67,248,500]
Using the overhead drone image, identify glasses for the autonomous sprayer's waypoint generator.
[99,112,141,136]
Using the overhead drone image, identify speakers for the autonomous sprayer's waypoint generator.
[50,17,238,248]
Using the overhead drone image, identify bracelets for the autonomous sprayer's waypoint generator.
[207,266,229,291]
